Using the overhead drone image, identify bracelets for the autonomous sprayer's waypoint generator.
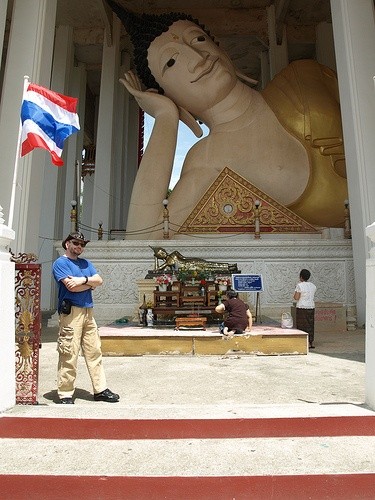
[82,275,88,285]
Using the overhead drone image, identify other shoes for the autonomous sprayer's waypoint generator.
[61,398,74,404]
[94,388,120,401]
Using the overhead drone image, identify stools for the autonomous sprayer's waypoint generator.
[151,279,232,332]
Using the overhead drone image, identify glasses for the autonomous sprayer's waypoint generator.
[70,241,85,247]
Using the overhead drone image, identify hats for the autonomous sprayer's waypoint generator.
[62,231,90,250]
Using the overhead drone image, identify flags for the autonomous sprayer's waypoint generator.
[19,82,80,166]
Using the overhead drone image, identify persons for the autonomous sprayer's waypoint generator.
[119,11,349,241]
[53,232,120,404]
[294,268,317,348]
[215,289,252,336]
[148,245,238,272]
